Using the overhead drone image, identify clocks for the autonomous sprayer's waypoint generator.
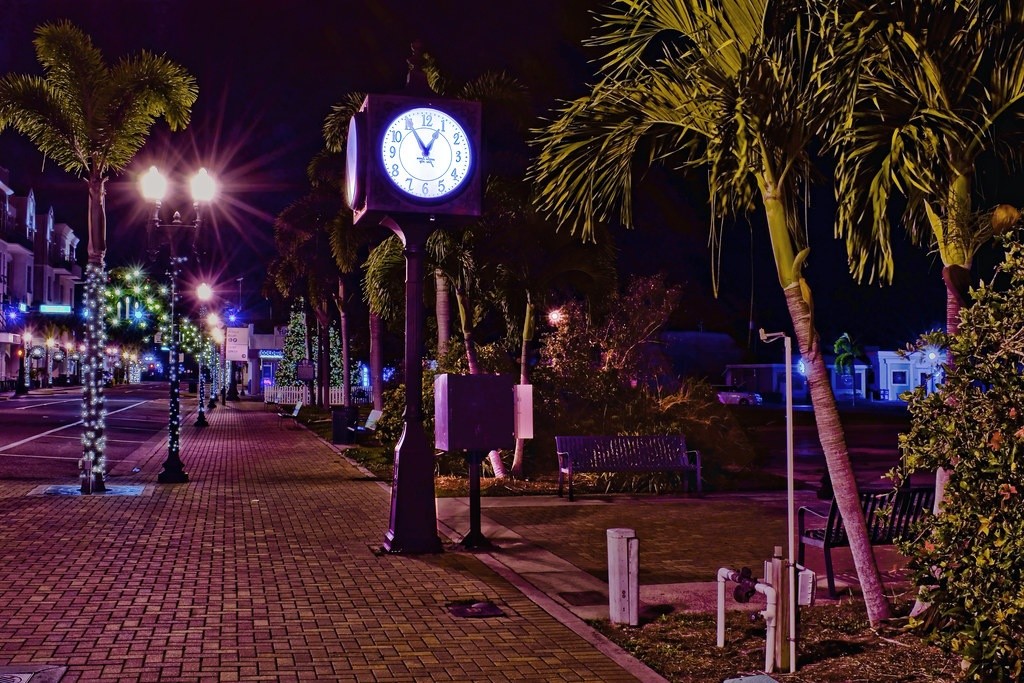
[379,105,474,204]
[346,111,364,212]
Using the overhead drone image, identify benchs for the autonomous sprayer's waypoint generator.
[347,410,383,444]
[555,435,705,501]
[797,482,935,599]
[277,401,303,430]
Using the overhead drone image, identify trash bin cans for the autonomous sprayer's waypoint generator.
[330,404,360,446]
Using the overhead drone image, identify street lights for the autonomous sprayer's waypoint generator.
[207,312,227,409]
[47,337,57,387]
[14,333,33,394]
[927,352,937,394]
[65,342,73,386]
[137,166,222,484]
[195,281,214,430]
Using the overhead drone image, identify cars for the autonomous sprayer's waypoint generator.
[709,384,762,409]
